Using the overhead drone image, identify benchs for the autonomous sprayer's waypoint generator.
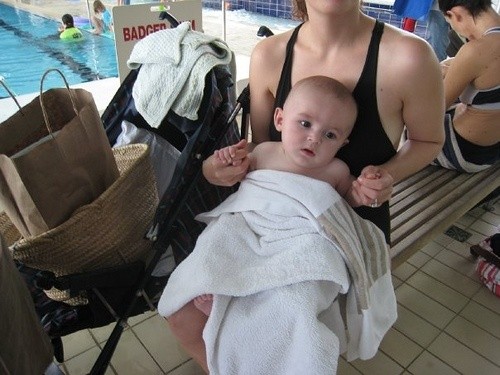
[389,165,500,273]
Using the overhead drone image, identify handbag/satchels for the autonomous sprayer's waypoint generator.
[0,69,122,239]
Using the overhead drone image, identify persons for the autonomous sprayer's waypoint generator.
[404,0,499,173]
[193,76,382,317]
[58,13,85,44]
[164,0,444,375]
[90,0,113,36]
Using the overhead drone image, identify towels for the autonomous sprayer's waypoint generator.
[158,169,398,375]
[126,21,232,128]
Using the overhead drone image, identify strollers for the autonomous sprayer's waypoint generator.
[14,11,275,375]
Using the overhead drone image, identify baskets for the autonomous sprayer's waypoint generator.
[0,143,159,307]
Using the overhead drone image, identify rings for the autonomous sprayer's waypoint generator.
[370,200,381,208]
